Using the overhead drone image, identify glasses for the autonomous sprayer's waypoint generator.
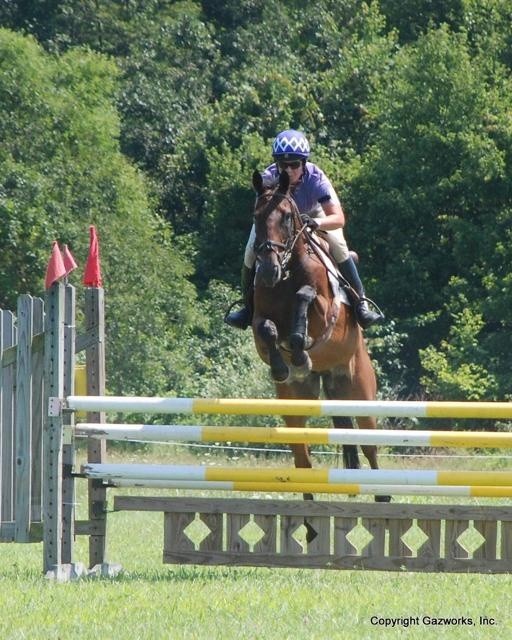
[277,160,302,169]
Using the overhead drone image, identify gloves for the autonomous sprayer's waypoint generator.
[300,212,318,232]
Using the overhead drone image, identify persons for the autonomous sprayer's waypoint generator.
[224,130,382,331]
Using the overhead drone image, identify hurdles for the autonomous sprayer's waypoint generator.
[37,279,512,586]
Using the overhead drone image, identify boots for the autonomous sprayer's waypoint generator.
[337,255,382,329]
[224,261,257,331]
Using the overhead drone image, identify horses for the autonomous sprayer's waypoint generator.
[250,168,393,503]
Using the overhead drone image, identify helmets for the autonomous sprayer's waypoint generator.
[272,129,311,158]
